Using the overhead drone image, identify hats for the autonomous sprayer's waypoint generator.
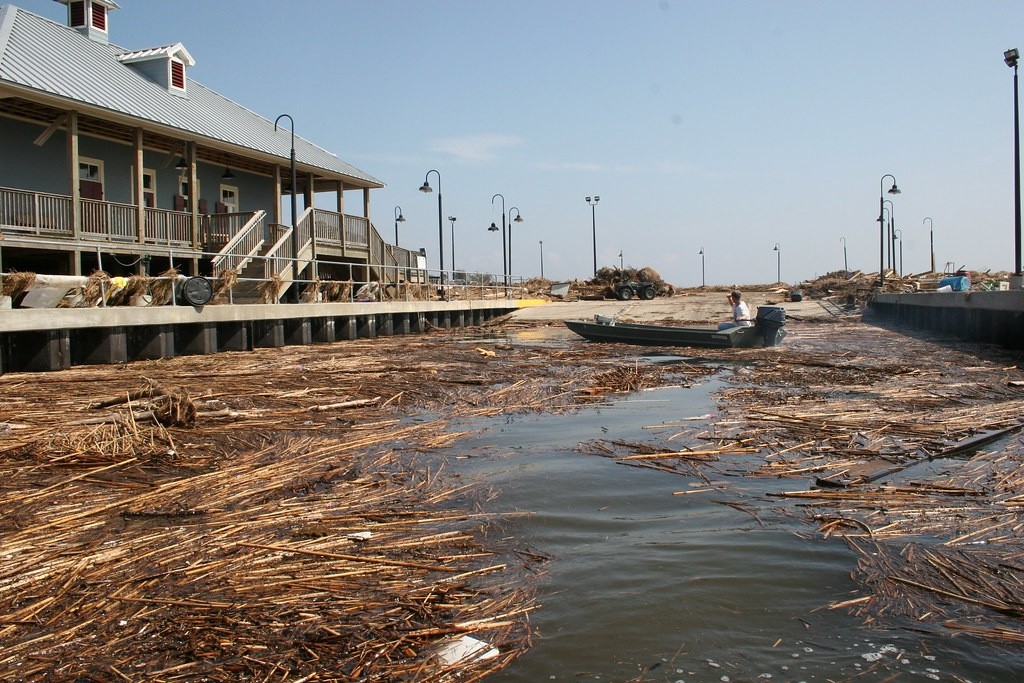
[730,290,741,298]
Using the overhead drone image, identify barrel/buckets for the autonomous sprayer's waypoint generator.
[939,276,971,291]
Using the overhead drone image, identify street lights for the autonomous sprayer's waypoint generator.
[619,250,623,270]
[394,206,405,247]
[508,206,524,285]
[922,217,934,274]
[585,194,599,277]
[1003,48,1023,277]
[699,247,705,287]
[486,193,507,297]
[447,216,456,281]
[419,168,448,302]
[876,208,890,270]
[892,229,902,277]
[773,243,780,282]
[880,174,902,287]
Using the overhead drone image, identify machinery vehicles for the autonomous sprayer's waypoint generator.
[612,275,657,301]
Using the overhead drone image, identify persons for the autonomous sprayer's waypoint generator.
[718,290,751,331]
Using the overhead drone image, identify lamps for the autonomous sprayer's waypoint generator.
[221,154,235,180]
[175,143,188,170]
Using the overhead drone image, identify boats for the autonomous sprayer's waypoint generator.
[563,303,788,350]
